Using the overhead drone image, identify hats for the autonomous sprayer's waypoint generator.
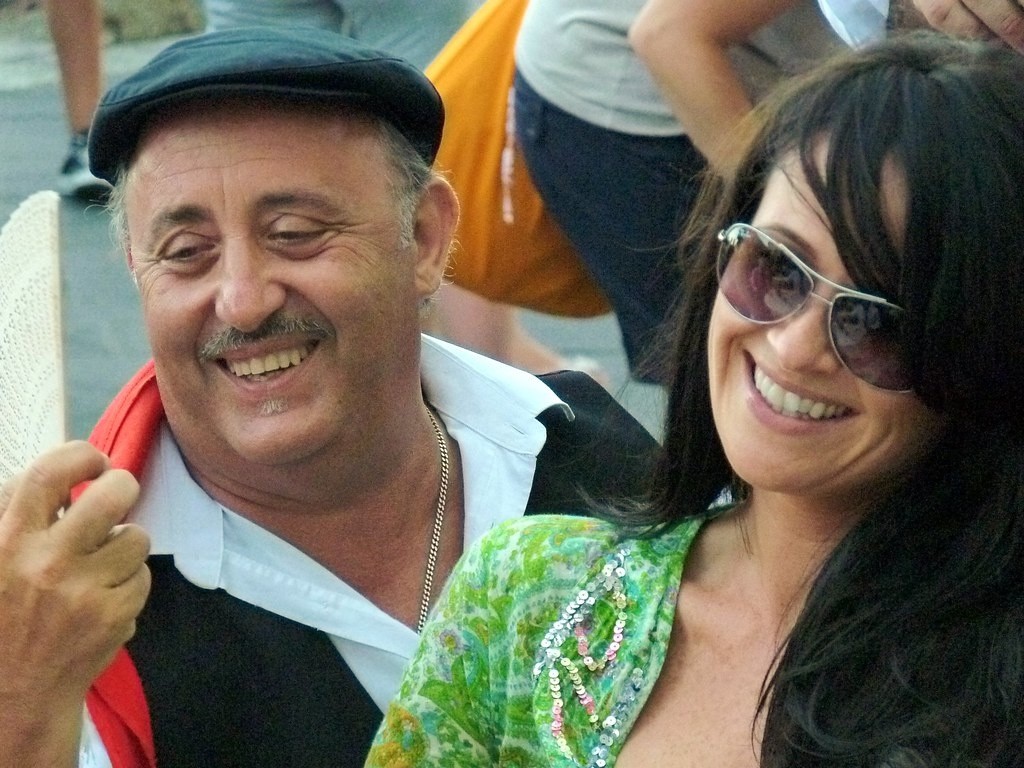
[86,27,446,190]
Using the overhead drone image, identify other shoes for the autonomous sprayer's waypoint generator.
[58,130,117,202]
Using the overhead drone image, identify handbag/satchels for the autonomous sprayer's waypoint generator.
[423,0,618,319]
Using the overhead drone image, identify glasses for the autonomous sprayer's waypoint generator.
[715,222,916,393]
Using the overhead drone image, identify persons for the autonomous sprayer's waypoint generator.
[47,0,114,207]
[750,256,802,309]
[834,298,902,349]
[511,0,826,389]
[362,28,1024,767]
[0,33,660,768]
[202,0,514,368]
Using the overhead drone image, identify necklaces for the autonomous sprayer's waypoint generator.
[417,405,448,635]
